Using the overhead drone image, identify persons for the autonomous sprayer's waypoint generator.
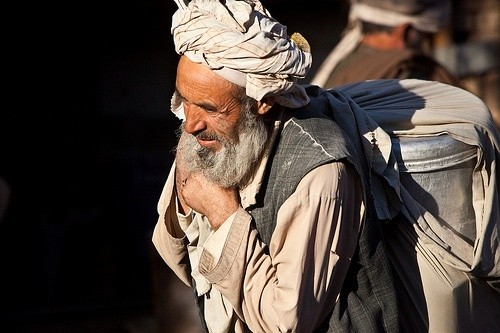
[150,0,398,333]
[304,1,500,134]
